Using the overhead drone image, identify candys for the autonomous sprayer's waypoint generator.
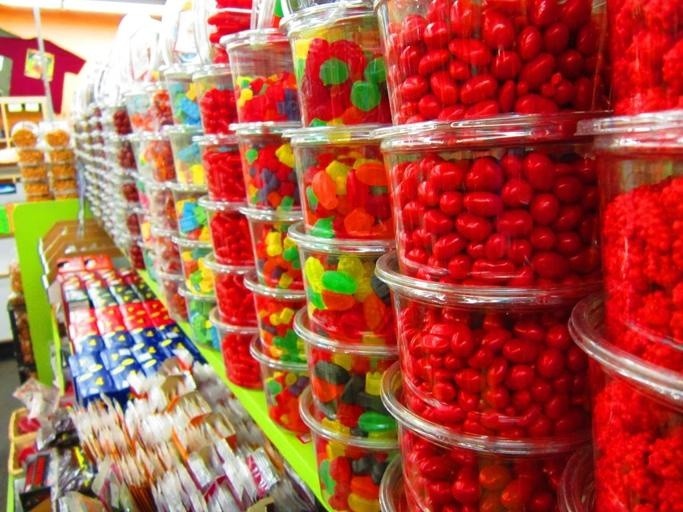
[12,130,81,201]
[76,0,683,512]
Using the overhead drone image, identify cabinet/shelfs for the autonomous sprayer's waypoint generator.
[0,96,49,195]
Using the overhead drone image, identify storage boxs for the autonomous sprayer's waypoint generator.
[6,407,38,512]
